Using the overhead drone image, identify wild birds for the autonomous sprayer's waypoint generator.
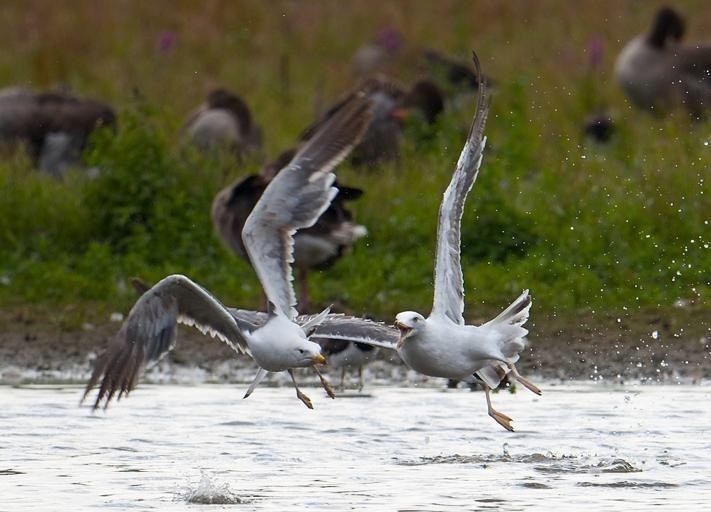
[129,48,548,434]
[209,143,372,314]
[322,332,380,400]
[0,80,118,179]
[341,73,444,176]
[612,4,710,133]
[79,80,380,412]
[167,88,269,173]
